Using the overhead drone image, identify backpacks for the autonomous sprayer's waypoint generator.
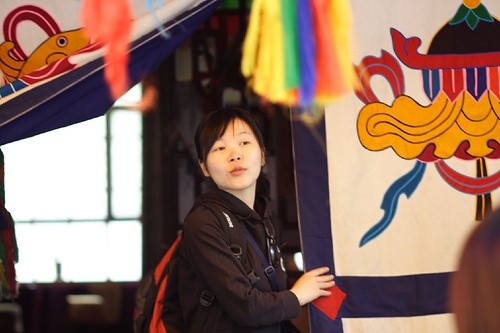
[134,200,261,333]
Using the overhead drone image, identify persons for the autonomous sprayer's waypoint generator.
[177,105,336,333]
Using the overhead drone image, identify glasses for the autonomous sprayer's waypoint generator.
[262,217,281,268]
